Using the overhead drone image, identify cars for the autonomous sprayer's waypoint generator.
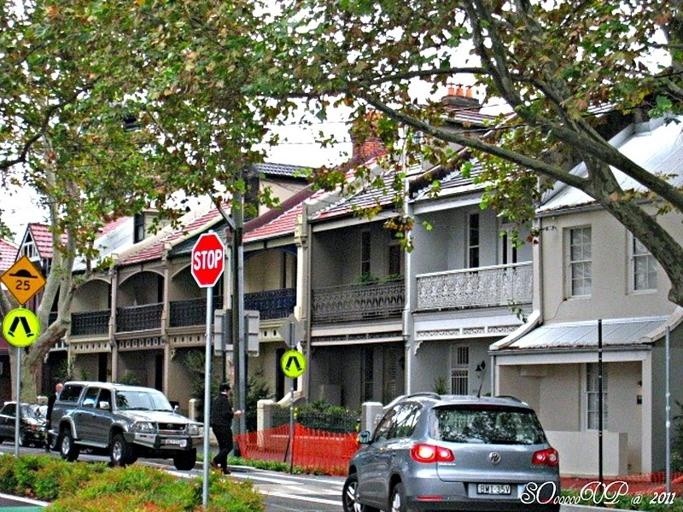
[0,401,47,446]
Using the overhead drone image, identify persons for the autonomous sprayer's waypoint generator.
[45,383,64,453]
[210,383,242,475]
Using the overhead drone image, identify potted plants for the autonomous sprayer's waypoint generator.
[351,271,404,321]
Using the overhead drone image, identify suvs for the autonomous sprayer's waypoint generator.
[49,380,204,470]
[343,390,560,512]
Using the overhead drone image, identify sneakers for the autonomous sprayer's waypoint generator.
[210,461,230,474]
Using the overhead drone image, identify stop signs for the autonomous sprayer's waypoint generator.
[191,232,225,288]
